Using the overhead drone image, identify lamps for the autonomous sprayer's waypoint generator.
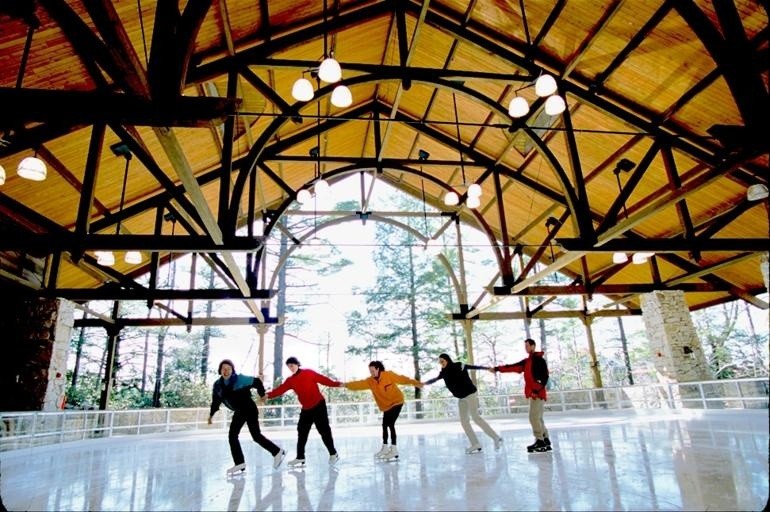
[612,159,655,265]
[747,172,769,201]
[546,217,564,285]
[292,50,352,108]
[297,147,328,204]
[508,0,567,118]
[445,93,481,209]
[412,150,441,256]
[0,0,47,186]
[94,139,142,266]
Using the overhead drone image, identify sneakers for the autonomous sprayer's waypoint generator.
[329,453,338,464]
[288,459,305,465]
[227,463,246,474]
[526,437,551,449]
[375,444,399,459]
[494,436,500,450]
[274,448,284,468]
[464,444,481,452]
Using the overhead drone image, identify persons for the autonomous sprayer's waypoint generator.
[423,354,505,455]
[493,339,552,454]
[340,360,424,458]
[208,359,285,474]
[261,357,344,468]
